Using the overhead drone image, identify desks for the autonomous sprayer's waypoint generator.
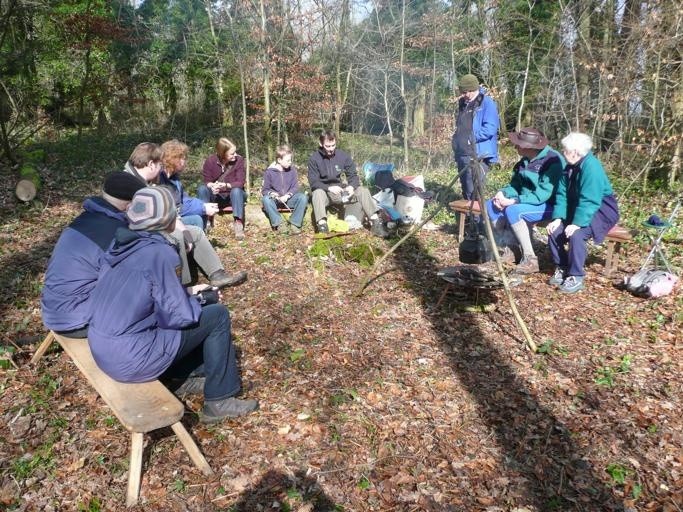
[641,220,674,274]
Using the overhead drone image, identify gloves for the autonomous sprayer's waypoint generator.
[199,286,220,305]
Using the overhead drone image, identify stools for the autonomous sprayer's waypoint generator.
[221,205,248,213]
[262,206,306,228]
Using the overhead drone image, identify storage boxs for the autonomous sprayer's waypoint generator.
[396,175,425,224]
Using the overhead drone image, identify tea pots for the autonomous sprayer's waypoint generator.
[396,215,416,226]
[459,222,492,264]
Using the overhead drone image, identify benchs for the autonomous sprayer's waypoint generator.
[31,327,213,507]
[449,199,632,275]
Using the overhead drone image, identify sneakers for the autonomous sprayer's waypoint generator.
[209,269,247,290]
[169,376,206,398]
[288,223,301,234]
[557,274,584,293]
[317,222,329,235]
[547,268,568,285]
[233,221,245,241]
[277,221,289,235]
[369,219,390,238]
[201,395,258,424]
[510,255,540,274]
[502,244,523,263]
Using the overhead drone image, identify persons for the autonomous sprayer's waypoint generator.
[88,187,259,423]
[546,131,620,292]
[197,137,249,240]
[156,139,220,231]
[262,145,309,235]
[450,74,498,225]
[478,127,567,275]
[124,143,248,289]
[41,170,144,340]
[307,131,390,239]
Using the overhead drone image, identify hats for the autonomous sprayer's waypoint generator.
[103,171,147,201]
[459,74,479,94]
[508,127,548,150]
[126,186,178,234]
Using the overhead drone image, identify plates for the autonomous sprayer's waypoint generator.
[641,221,672,228]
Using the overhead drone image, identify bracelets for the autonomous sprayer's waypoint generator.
[269,191,272,195]
[287,193,291,197]
[225,182,227,188]
